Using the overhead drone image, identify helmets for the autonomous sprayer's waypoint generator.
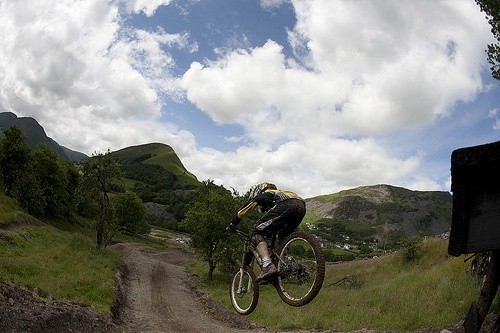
[253,182,277,197]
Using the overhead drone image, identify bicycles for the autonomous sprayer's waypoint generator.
[224,218,326,315]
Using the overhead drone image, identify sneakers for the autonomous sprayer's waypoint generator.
[256,263,277,280]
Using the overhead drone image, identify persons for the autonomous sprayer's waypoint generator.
[227,181,307,282]
